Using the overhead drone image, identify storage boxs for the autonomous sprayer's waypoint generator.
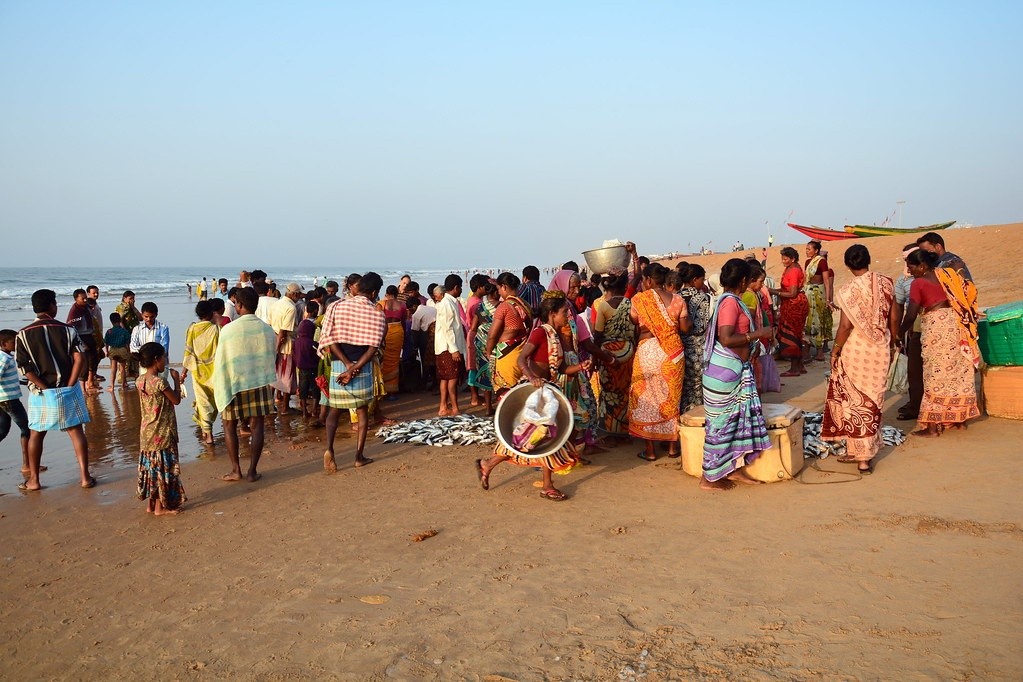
[978,365,1023,421]
[675,402,805,483]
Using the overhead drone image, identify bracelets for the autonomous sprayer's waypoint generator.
[890,347,894,351]
[829,351,839,359]
[633,257,640,263]
[745,333,751,343]
[833,341,840,348]
[609,357,615,365]
[633,252,637,256]
[895,334,903,340]
[778,292,781,296]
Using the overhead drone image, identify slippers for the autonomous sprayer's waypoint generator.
[668,450,680,458]
[309,419,326,426]
[540,489,568,500]
[837,454,857,463]
[476,459,489,490]
[82,476,97,488]
[638,451,656,461]
[860,461,872,475]
[18,481,40,491]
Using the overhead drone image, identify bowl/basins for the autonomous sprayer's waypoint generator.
[494,382,574,459]
[581,245,631,274]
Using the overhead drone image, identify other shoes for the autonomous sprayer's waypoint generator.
[39,465,47,470]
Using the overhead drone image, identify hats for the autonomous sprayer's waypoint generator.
[744,254,755,258]
[708,274,721,291]
[287,283,301,293]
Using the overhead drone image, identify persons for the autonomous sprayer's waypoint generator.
[0,329,49,473]
[464,241,642,500]
[375,273,469,416]
[132,341,188,516]
[178,268,384,482]
[627,241,842,491]
[819,232,988,475]
[13,289,97,490]
[768,234,775,247]
[66,284,170,394]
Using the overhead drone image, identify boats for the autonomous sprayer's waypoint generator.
[787,222,858,243]
[843,220,958,238]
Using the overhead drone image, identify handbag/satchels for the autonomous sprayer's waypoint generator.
[885,341,911,394]
[759,338,781,392]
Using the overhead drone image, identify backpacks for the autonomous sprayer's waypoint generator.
[512,422,558,453]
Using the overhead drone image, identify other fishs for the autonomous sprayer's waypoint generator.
[374,412,498,448]
[802,409,906,461]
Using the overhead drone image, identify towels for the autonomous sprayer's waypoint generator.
[213,314,278,413]
